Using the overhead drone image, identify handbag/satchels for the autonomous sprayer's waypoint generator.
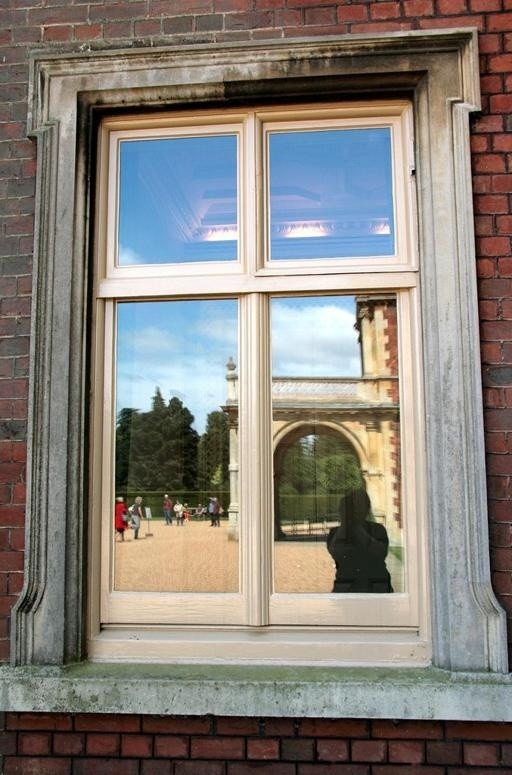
[121,514,130,522]
[218,506,224,514]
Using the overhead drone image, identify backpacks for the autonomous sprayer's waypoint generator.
[127,504,135,513]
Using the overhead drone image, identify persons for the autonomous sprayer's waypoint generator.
[325,487,393,593]
[114,494,223,542]
